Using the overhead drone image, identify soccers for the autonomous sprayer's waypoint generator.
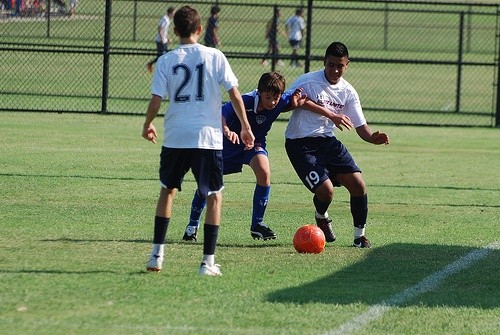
[293,223,328,255]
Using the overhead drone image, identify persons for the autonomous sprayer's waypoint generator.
[0,0,79,19]
[204,6,224,49]
[146,6,177,72]
[141,5,256,278]
[259,3,306,71]
[285,40,390,248]
[181,70,308,242]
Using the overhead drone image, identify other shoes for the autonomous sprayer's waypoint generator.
[290,64,295,67]
[276,59,285,66]
[295,61,304,66]
[262,61,269,65]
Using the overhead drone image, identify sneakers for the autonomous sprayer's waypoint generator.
[145,63,153,72]
[249,223,277,241]
[353,236,372,249]
[315,211,336,242]
[146,254,163,273]
[182,225,198,245]
[199,262,222,277]
